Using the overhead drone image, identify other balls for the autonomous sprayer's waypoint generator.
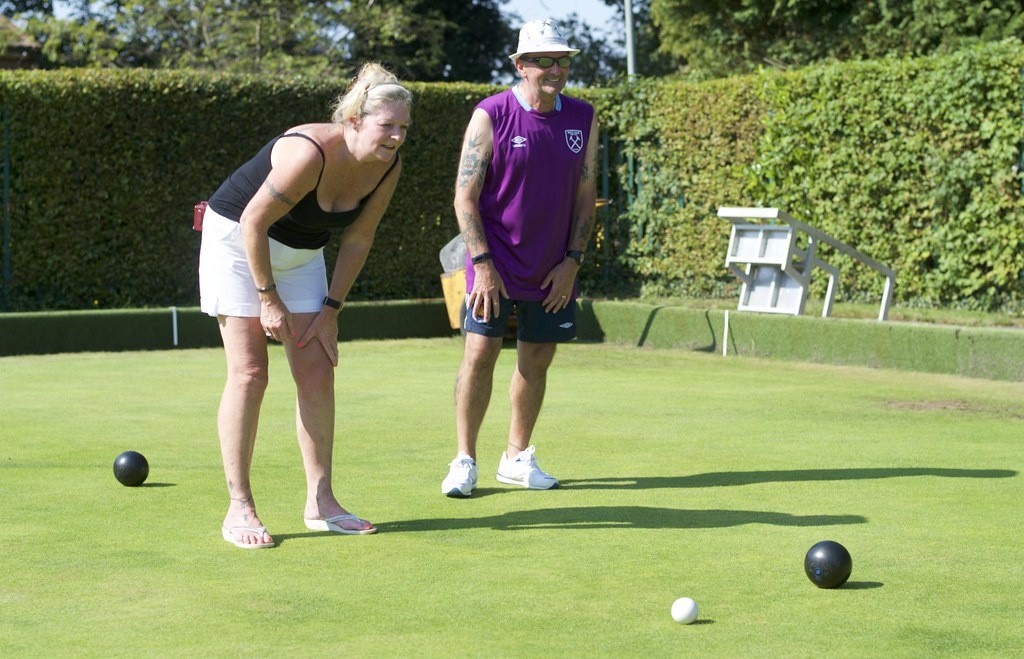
[113,451,149,485]
[671,597,699,624]
[804,540,852,588]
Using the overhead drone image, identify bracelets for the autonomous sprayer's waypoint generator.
[257,283,277,293]
[323,297,344,311]
[471,252,493,265]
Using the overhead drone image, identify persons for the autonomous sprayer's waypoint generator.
[197,61,413,552]
[440,18,600,495]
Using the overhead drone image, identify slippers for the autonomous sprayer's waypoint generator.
[223,524,274,549]
[304,515,377,535]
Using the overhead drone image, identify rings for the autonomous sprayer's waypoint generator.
[561,295,566,300]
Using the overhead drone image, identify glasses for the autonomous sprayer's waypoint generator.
[521,56,573,68]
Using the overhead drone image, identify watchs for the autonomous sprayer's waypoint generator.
[564,250,586,265]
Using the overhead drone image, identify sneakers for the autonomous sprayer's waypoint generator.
[496,446,558,489]
[442,455,478,495]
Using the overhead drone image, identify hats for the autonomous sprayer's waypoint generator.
[509,18,581,61]
